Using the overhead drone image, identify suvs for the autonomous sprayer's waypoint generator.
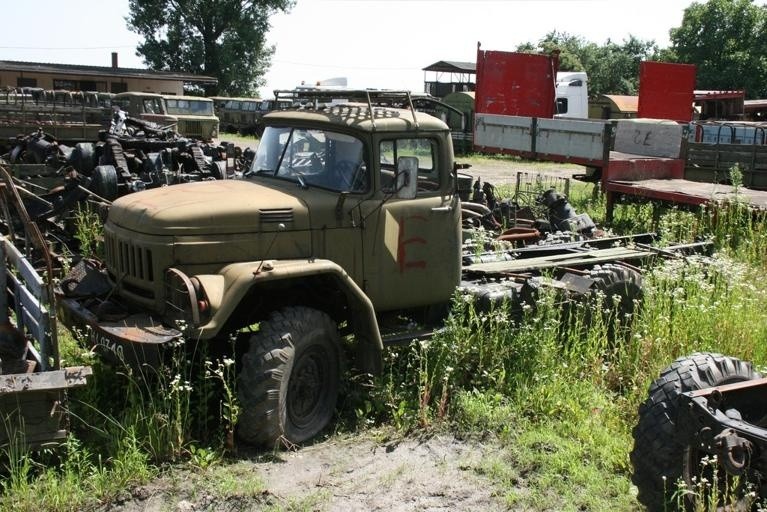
[628,351,767,512]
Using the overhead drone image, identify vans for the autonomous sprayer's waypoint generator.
[0,85,291,142]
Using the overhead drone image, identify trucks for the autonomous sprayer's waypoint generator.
[56,100,716,453]
[554,71,589,118]
